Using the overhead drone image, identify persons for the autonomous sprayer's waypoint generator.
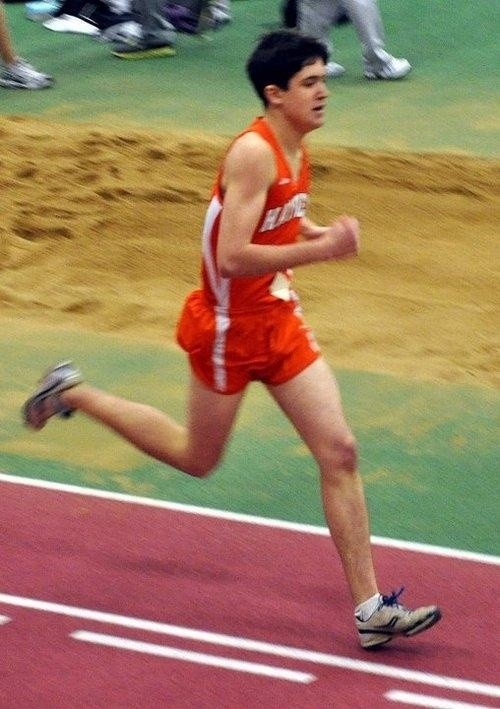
[0,0,411,91]
[16,27,448,652]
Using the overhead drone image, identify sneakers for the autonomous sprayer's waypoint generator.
[43,14,100,38]
[110,40,178,63]
[21,360,84,432]
[355,602,441,651]
[0,59,58,91]
[318,55,413,81]
